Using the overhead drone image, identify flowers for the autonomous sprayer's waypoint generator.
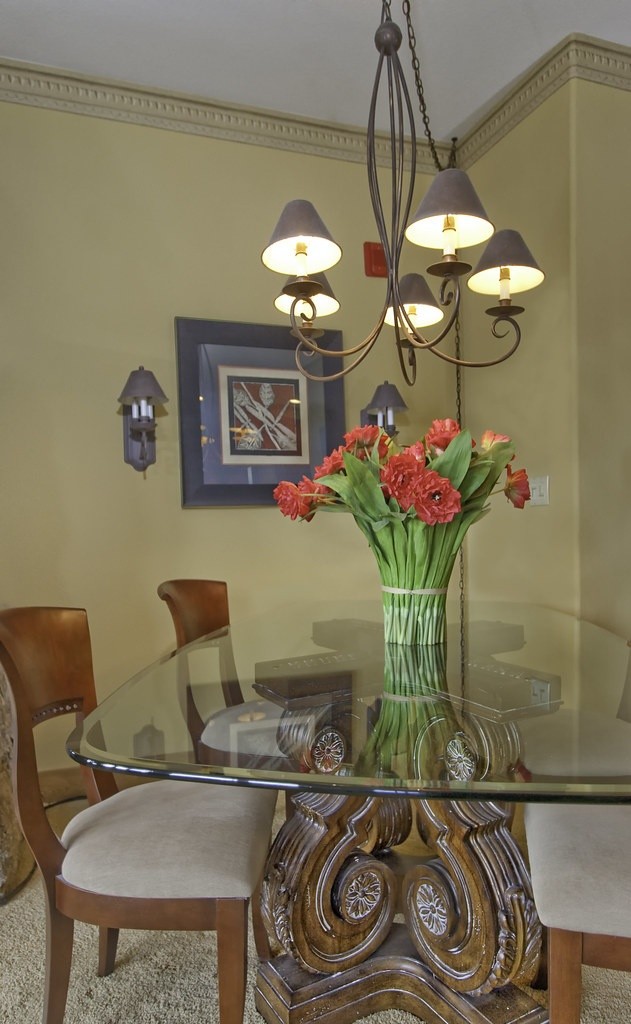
[273,419,531,645]
[353,645,532,787]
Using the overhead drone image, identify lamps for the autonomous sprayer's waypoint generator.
[360,380,407,440]
[117,366,170,472]
[237,705,266,722]
[261,1,546,385]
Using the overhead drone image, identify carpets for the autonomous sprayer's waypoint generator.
[0,862,631,1024]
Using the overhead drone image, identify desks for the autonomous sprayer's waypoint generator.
[65,603,631,1024]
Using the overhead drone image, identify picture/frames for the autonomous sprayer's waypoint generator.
[174,317,345,509]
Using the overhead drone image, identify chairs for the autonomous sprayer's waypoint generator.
[522,802,631,1024]
[158,579,333,820]
[0,607,279,1024]
